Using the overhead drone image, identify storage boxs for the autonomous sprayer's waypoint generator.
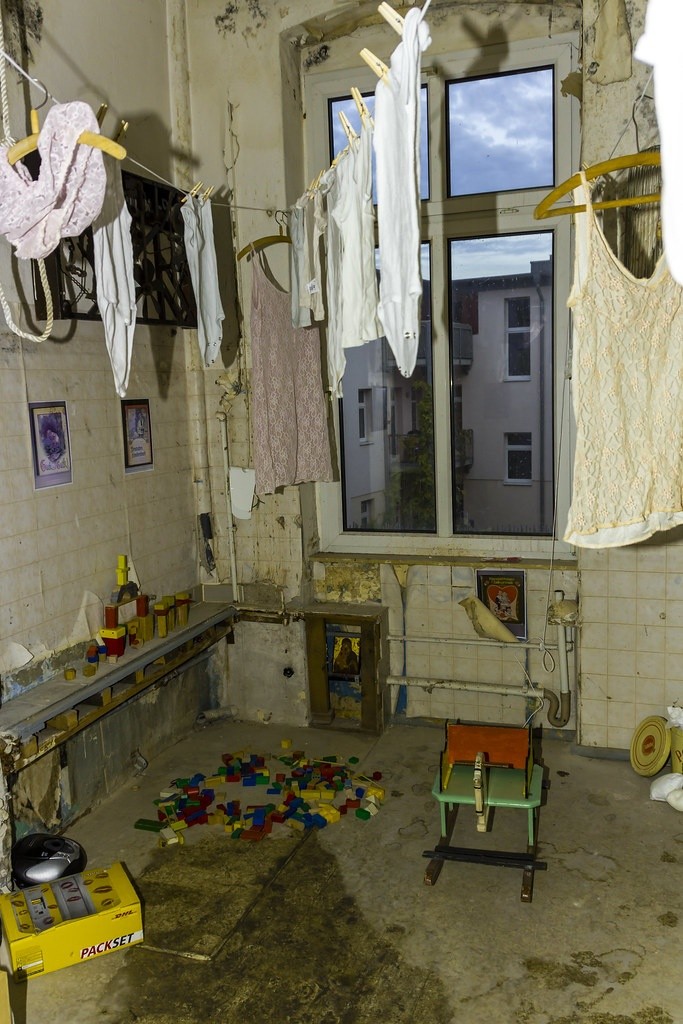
[0,862,143,984]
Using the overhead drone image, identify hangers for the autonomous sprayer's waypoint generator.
[533,92,661,220]
[5,77,127,166]
[236,210,292,262]
